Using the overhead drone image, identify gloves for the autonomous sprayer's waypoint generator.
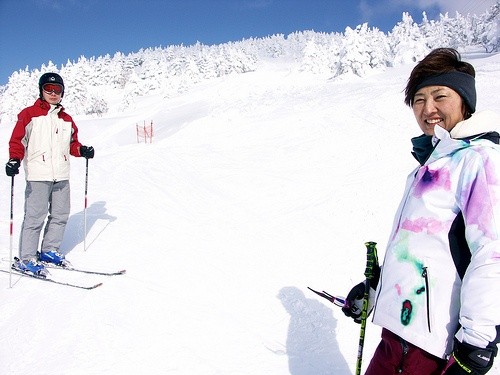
[6,159,20,176]
[80,146,94,158]
[341,280,377,323]
[440,338,498,375]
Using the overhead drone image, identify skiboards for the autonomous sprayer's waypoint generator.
[0,249,126,291]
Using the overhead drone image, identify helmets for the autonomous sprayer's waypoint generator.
[39,73,64,98]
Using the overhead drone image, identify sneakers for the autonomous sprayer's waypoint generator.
[37,251,65,267]
[14,259,45,276]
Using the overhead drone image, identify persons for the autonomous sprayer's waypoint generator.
[341,47,500,375]
[5,72,95,278]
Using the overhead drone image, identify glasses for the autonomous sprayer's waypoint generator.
[41,83,63,94]
